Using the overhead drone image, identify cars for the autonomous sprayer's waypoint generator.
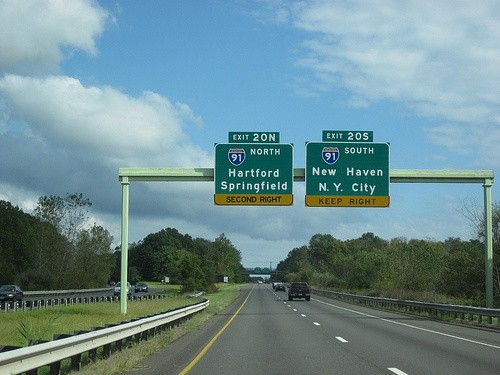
[287,282,311,301]
[274,284,286,292]
[114,282,132,296]
[256,280,266,284]
[0,285,24,302]
[134,283,149,294]
[109,280,116,286]
[272,282,282,289]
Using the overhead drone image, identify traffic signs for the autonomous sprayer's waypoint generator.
[303,139,392,208]
[212,142,294,207]
